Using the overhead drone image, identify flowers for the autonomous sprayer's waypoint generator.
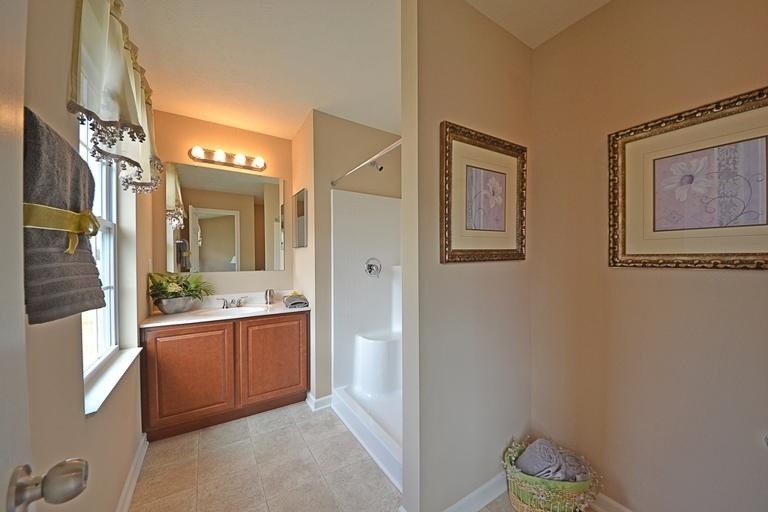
[467,172,504,230]
[145,268,213,299]
[659,156,765,228]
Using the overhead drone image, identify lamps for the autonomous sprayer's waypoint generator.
[370,161,383,172]
[186,144,267,173]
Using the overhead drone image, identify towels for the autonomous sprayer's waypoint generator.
[25,109,107,324]
[282,294,312,309]
[515,437,585,478]
[176,241,191,267]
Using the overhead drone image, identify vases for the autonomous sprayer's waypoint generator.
[153,293,199,314]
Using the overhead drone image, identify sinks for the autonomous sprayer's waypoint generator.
[195,308,264,315]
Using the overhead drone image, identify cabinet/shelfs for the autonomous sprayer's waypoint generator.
[143,308,308,441]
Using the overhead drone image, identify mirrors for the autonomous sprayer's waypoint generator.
[164,160,286,274]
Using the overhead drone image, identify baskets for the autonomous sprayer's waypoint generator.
[500,435,603,511]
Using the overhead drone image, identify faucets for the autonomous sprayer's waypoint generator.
[230,299,236,307]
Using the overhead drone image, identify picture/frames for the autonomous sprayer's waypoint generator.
[436,119,531,267]
[605,82,768,270]
[290,187,307,249]
